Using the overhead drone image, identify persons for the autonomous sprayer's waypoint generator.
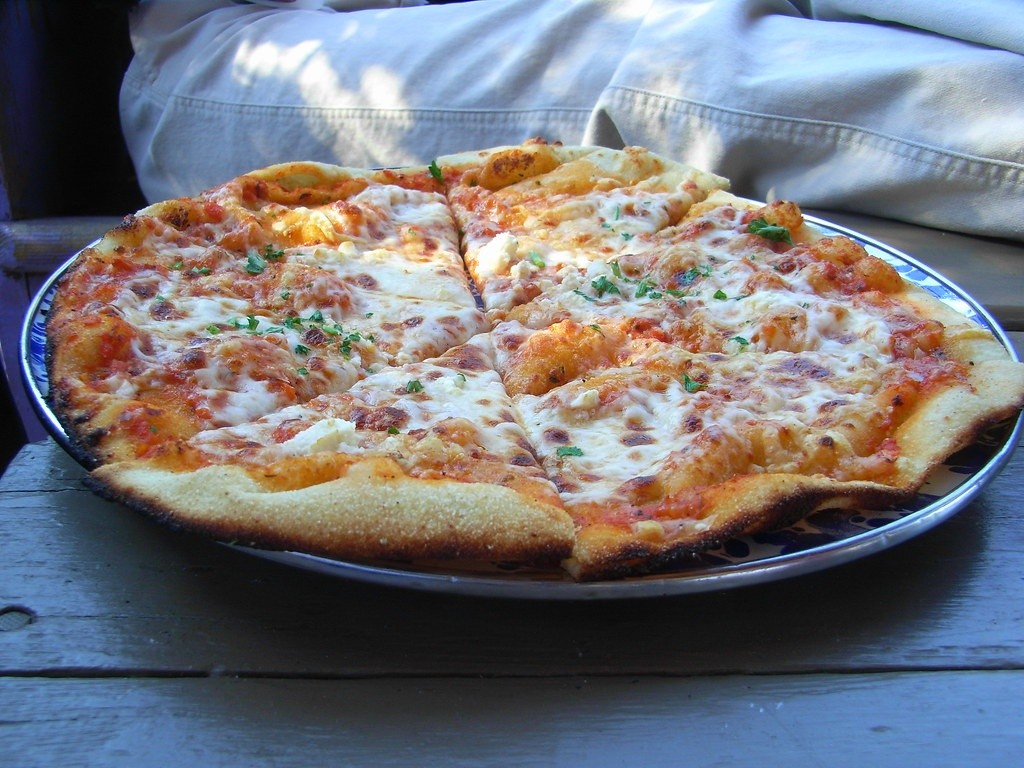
[118,1,1024,250]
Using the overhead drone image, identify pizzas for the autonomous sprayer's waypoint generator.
[44,138,1024,582]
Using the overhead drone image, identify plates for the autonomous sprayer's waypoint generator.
[19,199,1024,599]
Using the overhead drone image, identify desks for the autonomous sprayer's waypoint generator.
[0,212,1024,768]
